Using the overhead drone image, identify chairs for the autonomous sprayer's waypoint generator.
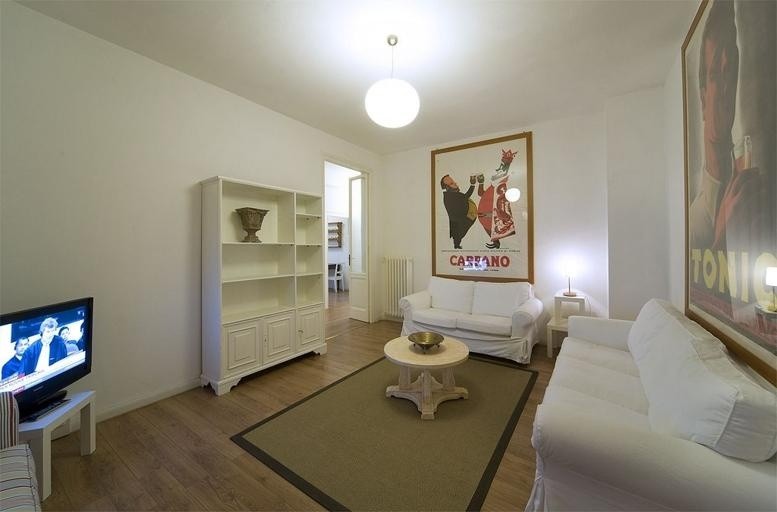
[328,262,344,293]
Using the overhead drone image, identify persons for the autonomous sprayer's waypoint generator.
[440,174,477,250]
[477,148,519,250]
[59,326,77,355]
[2,335,29,379]
[690,0,761,283]
[76,321,86,351]
[22,317,68,376]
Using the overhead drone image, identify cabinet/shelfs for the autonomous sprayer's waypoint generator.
[199,176,327,396]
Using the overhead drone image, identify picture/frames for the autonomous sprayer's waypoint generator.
[680,1,776,387]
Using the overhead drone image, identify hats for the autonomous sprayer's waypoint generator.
[501,148,518,163]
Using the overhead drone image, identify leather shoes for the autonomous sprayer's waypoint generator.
[486,243,500,248]
[454,244,461,249]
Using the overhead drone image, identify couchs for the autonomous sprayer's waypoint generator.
[1,391,41,512]
[522,298,777,512]
[398,276,543,365]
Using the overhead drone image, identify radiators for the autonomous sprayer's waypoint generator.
[383,256,412,318]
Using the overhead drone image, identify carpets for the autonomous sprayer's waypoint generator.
[228,354,539,512]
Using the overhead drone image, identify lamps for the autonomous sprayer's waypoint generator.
[504,188,520,202]
[765,267,777,311]
[560,254,578,297]
[365,35,421,128]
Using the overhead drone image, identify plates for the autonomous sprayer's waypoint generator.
[407,332,444,350]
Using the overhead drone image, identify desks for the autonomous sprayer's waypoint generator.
[18,390,97,502]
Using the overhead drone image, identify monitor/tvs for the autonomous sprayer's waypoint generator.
[0,296,93,419]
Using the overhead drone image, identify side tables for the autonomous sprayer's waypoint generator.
[754,300,777,346]
[546,293,587,359]
